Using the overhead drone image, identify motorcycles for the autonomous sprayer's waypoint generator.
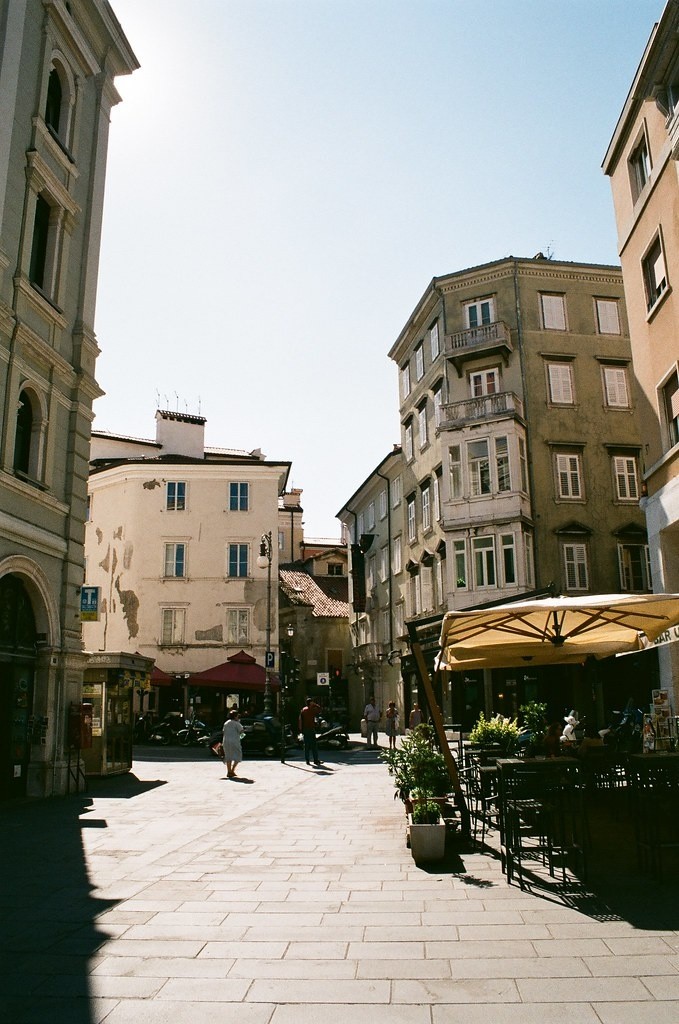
[297,716,350,750]
[176,712,212,747]
[135,713,172,746]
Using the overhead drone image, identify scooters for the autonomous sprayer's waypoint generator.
[609,706,651,753]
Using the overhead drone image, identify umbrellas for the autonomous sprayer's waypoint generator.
[404,583,679,838]
[135,651,172,687]
[188,650,281,703]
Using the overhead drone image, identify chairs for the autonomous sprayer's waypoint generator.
[453,742,679,891]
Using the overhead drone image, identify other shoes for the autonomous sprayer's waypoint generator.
[306,763,311,765]
[316,761,323,765]
[228,772,236,777]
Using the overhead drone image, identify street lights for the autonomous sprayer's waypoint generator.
[257,531,273,715]
[286,623,295,653]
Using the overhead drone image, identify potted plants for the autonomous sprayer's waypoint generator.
[378,722,453,864]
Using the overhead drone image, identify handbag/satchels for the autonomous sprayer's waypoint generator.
[212,742,226,760]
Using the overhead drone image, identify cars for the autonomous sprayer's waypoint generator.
[209,715,288,757]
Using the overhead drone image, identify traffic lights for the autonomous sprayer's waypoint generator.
[281,652,300,684]
[335,670,341,681]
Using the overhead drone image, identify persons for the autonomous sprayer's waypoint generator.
[222,710,244,778]
[386,701,399,749]
[299,698,324,764]
[543,723,563,754]
[410,703,425,730]
[229,703,239,711]
[563,716,578,740]
[364,697,382,749]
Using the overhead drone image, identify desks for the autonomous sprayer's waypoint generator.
[630,752,679,841]
[496,756,593,850]
[465,749,503,798]
